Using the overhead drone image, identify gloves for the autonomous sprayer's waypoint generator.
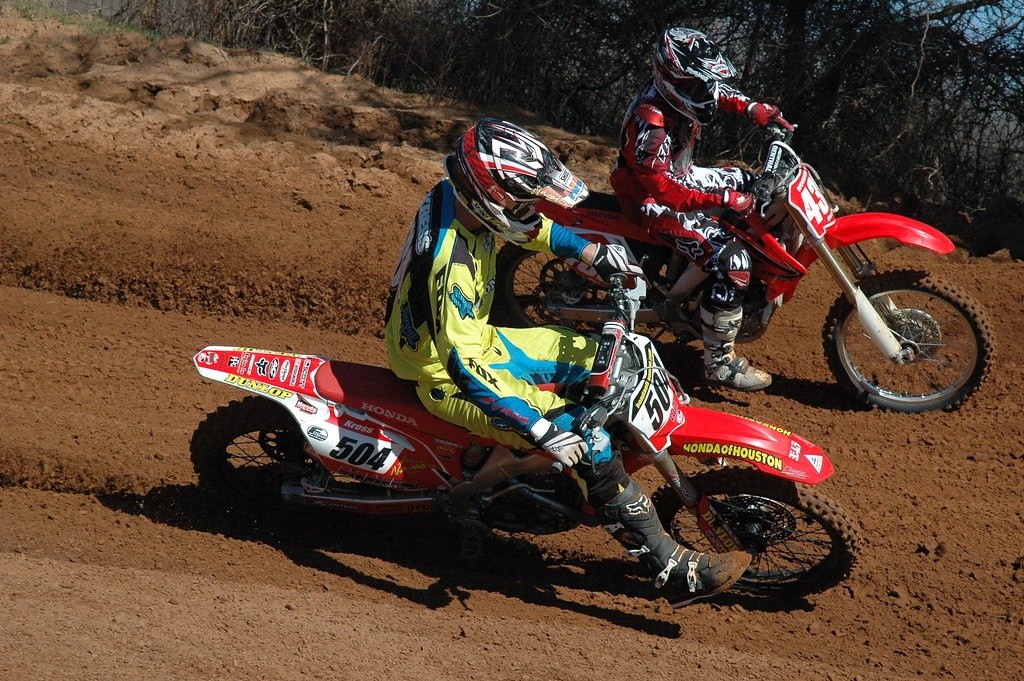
[749,102,794,132]
[723,186,757,220]
[535,423,588,469]
[590,242,643,283]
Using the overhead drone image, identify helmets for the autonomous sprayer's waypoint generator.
[443,119,590,246]
[653,27,739,125]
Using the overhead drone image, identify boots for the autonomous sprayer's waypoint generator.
[700,306,772,391]
[596,477,752,609]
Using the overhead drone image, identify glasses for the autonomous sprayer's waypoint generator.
[509,202,541,221]
[677,74,716,97]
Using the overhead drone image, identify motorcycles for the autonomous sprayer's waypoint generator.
[492,102,996,415]
[186,244,862,602]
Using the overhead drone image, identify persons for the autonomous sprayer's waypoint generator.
[385,119,752,609]
[611,27,792,393]
